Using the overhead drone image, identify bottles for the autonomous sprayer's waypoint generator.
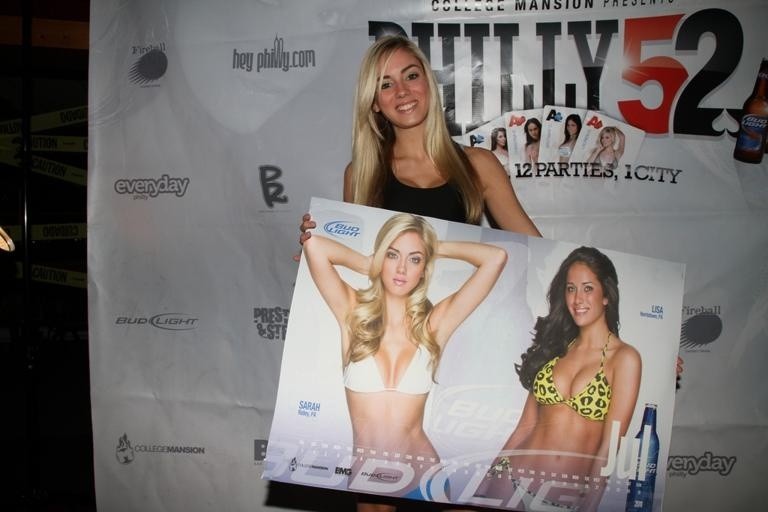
[622,400,662,512]
[728,55,767,167]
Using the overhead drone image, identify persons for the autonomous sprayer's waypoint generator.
[583,125,625,180]
[303,212,508,502]
[524,117,541,176]
[292,37,543,511]
[558,113,581,175]
[489,127,511,179]
[466,245,642,512]
